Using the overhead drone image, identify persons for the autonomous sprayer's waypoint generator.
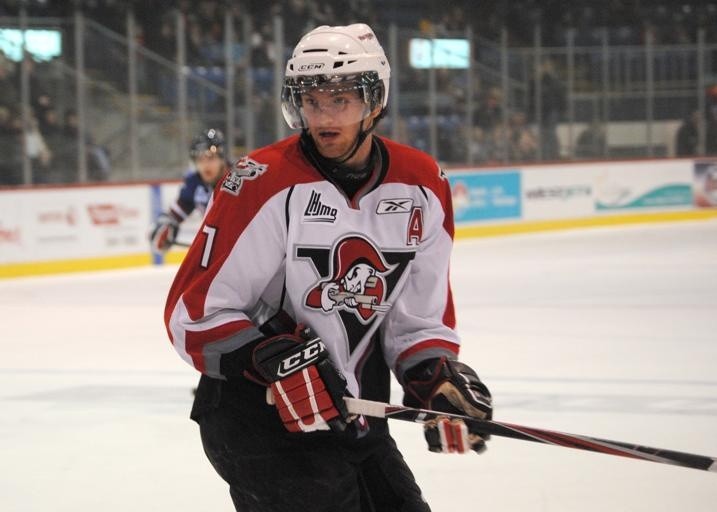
[163,23,492,512]
[1,0,717,189]
[150,129,236,254]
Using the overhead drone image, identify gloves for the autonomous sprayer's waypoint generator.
[148,212,179,252]
[243,319,370,442]
[401,355,494,452]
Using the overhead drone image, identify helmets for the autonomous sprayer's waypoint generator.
[188,128,226,159]
[280,21,392,109]
